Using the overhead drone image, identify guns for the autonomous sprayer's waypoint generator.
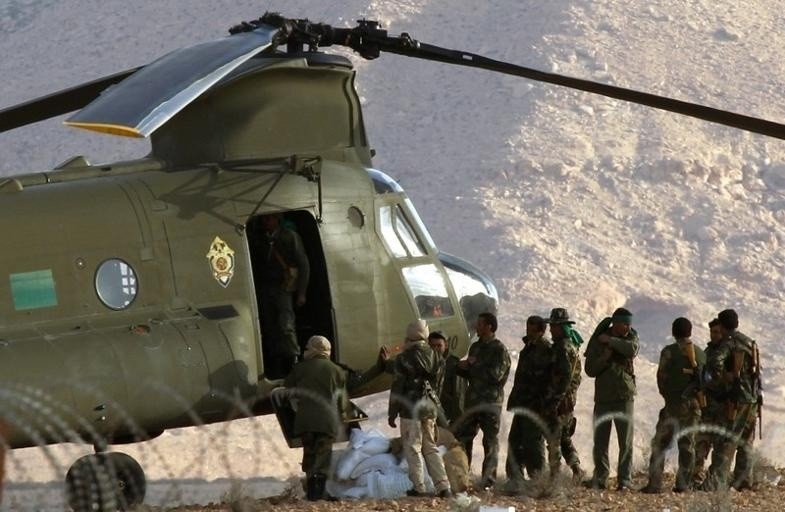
[714,351,745,443]
[751,339,762,438]
[682,338,707,408]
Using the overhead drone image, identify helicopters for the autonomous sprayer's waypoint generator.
[2,12,785,511]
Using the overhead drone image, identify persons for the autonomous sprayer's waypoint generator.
[283,335,348,501]
[253,214,310,373]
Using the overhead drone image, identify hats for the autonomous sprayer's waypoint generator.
[542,308,576,324]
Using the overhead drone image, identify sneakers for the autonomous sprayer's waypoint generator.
[673,485,690,493]
[582,478,604,489]
[437,489,452,498]
[406,489,419,496]
[637,484,664,494]
[616,483,628,491]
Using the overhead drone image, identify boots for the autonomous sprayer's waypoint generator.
[308,473,336,502]
[570,462,585,486]
